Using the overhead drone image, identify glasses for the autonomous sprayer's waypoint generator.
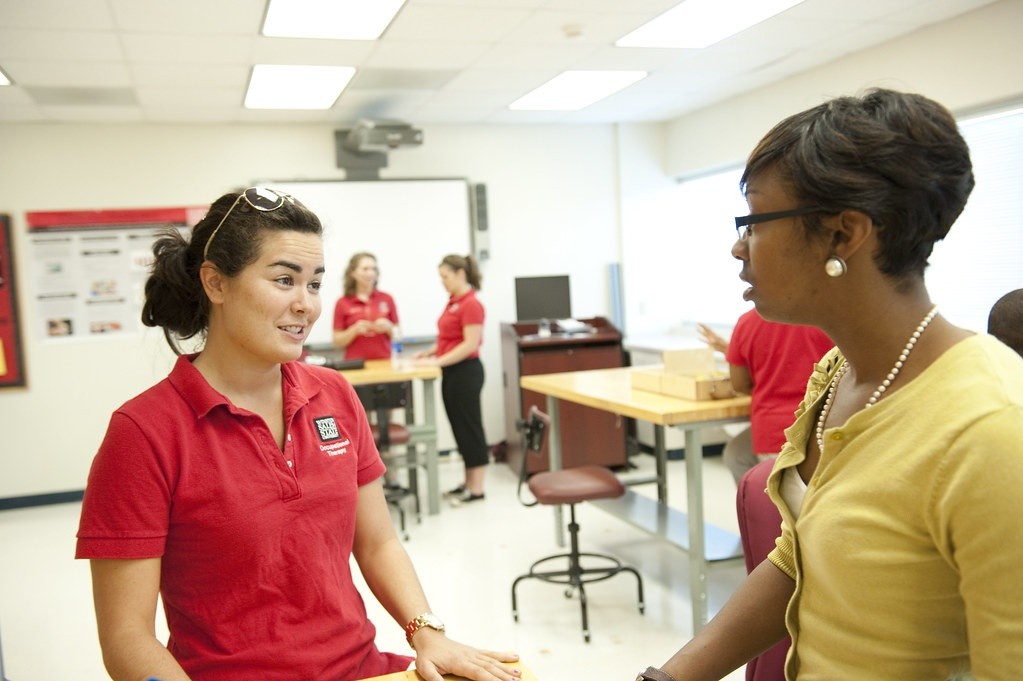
[734,203,883,244]
[204,187,307,259]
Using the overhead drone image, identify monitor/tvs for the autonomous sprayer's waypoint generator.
[515,276,571,340]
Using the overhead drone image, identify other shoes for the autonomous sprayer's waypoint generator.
[452,493,485,505]
[383,482,408,494]
[442,484,466,499]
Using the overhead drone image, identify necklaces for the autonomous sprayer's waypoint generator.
[816,305,939,456]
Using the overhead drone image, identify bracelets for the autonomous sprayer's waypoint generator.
[635,666,675,681]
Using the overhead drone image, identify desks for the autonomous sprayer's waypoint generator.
[337,354,441,517]
[519,363,755,638]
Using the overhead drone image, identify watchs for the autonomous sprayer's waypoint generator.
[404,612,446,650]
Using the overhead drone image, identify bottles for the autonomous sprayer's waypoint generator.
[390,325,402,364]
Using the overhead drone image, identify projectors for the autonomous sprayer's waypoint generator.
[343,127,422,152]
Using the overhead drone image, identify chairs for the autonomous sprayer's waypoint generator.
[361,382,421,540]
[510,407,645,645]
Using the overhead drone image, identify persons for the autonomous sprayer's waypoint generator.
[634,86,1023,681]
[726,305,836,464]
[74,189,523,681]
[694,323,759,482]
[331,253,399,427]
[412,254,490,505]
